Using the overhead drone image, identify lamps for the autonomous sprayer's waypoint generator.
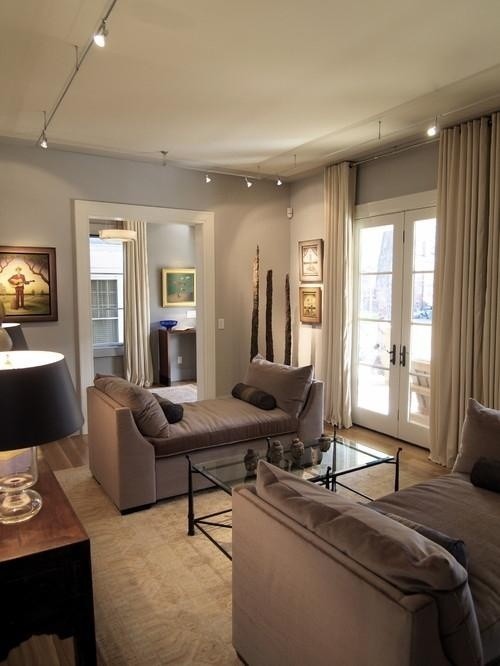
[0,350,86,524]
[244,175,253,188]
[205,174,211,183]
[93,23,110,46]
[99,222,136,242]
[276,175,282,186]
[1,323,30,350]
[427,116,438,136]
[39,131,49,148]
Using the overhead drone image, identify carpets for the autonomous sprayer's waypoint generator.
[1,453,500,666]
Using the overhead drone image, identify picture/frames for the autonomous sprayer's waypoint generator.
[162,268,198,307]
[297,239,323,282]
[0,246,58,323]
[298,287,322,323]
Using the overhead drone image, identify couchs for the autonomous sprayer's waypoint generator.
[86,353,324,516]
[229,471,500,666]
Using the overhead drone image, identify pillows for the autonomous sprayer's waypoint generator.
[245,354,315,417]
[450,397,500,474]
[231,382,278,409]
[152,392,184,424]
[256,458,483,666]
[93,372,172,439]
